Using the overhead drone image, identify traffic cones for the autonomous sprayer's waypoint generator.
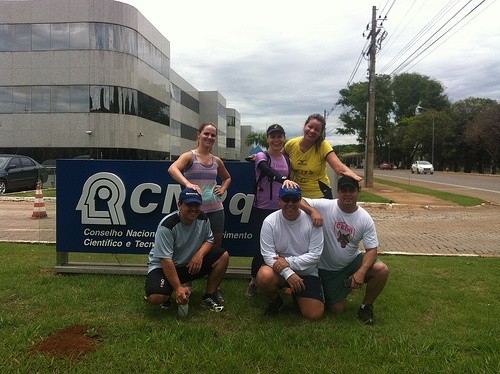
[28,184,48,220]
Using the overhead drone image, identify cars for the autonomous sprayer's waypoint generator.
[410,161,434,175]
[0,154,49,195]
[380,162,393,170]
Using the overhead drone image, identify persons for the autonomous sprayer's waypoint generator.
[168,122,232,304]
[244,124,300,299]
[252,113,362,199]
[251,186,325,320]
[297,176,389,323]
[144,188,229,312]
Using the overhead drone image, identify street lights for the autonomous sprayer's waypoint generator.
[378,125,390,163]
[417,106,434,166]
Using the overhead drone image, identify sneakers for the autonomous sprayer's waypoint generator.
[160,299,171,310]
[245,281,257,298]
[199,292,225,312]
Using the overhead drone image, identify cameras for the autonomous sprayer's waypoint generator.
[344,279,351,288]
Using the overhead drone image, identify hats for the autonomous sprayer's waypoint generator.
[179,189,202,206]
[267,124,285,136]
[279,186,301,199]
[338,176,359,188]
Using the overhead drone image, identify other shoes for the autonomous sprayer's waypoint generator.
[357,304,373,325]
[214,291,224,303]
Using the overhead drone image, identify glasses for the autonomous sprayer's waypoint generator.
[281,197,300,203]
